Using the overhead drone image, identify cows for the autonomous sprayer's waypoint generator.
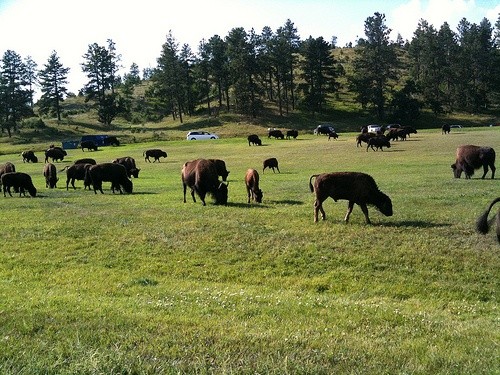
[248,134,262,146]
[450,145,496,179]
[316,125,339,139]
[355,125,417,152]
[286,130,298,140]
[143,149,167,163]
[207,159,230,181]
[43,163,59,189]
[103,136,120,147]
[42,144,67,164]
[268,130,284,139]
[263,158,280,174]
[476,197,500,245]
[81,141,97,152]
[245,168,263,203]
[22,150,38,163]
[0,162,36,198]
[60,156,141,195]
[441,124,450,135]
[309,171,393,224]
[181,158,228,206]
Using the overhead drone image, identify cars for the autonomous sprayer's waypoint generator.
[186,131,220,141]
[367,124,381,133]
[387,124,401,129]
